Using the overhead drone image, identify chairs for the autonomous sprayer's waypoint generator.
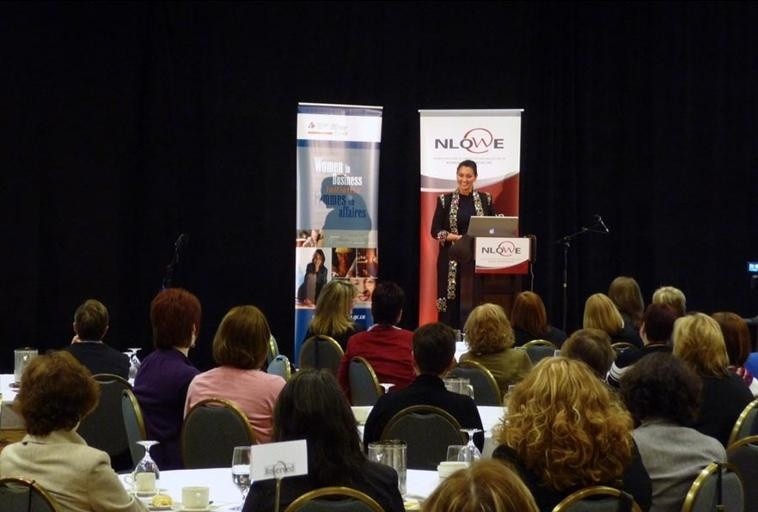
[725,435,758,512]
[447,357,504,409]
[175,395,259,473]
[283,484,385,512]
[520,339,556,364]
[0,475,62,512]
[297,334,346,384]
[73,372,137,474]
[378,404,470,471]
[726,396,758,454]
[345,354,387,407]
[120,386,153,475]
[610,342,635,356]
[551,482,643,512]
[267,354,292,382]
[681,462,748,512]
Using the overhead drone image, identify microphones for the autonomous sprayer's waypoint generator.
[596,216,610,233]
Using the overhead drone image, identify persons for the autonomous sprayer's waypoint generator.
[671,311,755,448]
[709,311,758,397]
[302,280,363,352]
[609,277,644,330]
[183,306,287,444]
[420,459,540,512]
[605,314,674,388]
[363,324,485,459]
[338,282,416,405]
[582,293,642,349]
[308,229,324,246]
[297,230,310,247]
[297,250,327,307]
[561,328,615,379]
[349,278,376,304]
[511,291,563,349]
[430,160,504,329]
[460,303,533,405]
[68,301,131,380]
[359,248,377,275]
[332,247,356,278]
[133,289,201,469]
[491,358,653,512]
[0,350,150,512]
[242,369,407,512]
[618,352,727,511]
[652,286,686,316]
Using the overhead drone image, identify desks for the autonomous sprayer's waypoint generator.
[0,373,27,443]
[115,468,467,511]
[350,404,512,455]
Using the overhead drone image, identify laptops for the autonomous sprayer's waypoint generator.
[467,216,518,237]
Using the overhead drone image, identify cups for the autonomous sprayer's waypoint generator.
[123,351,138,381]
[446,444,471,462]
[127,347,140,366]
[135,438,161,482]
[437,460,468,490]
[14,349,37,384]
[231,444,252,505]
[124,472,157,491]
[457,427,482,463]
[181,487,209,506]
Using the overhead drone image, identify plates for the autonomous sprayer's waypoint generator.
[7,382,21,391]
[132,488,168,496]
[179,506,210,511]
